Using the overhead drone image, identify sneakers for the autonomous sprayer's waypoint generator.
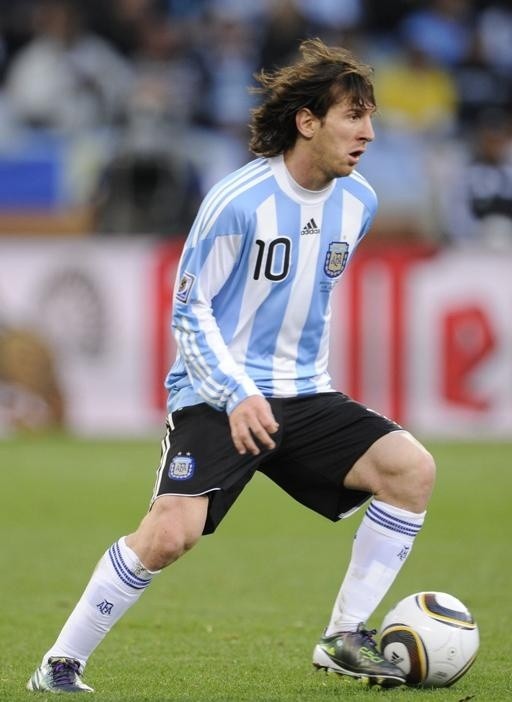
[310,621,410,687]
[25,656,94,694]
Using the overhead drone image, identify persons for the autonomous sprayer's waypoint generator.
[15,39,439,695]
[1,1,511,249]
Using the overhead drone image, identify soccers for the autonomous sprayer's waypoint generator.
[379,591,480,688]
